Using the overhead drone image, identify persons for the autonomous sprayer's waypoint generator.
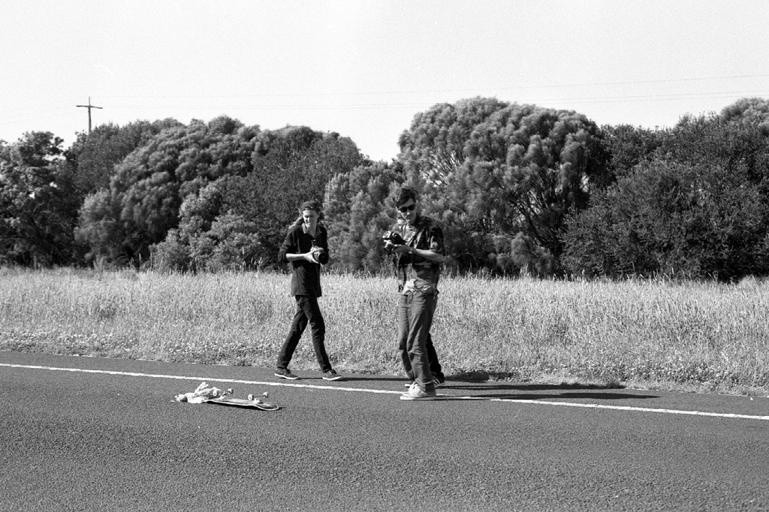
[272,199,344,381]
[426,331,446,385]
[382,188,447,401]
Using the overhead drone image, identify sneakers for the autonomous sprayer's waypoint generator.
[275,368,299,380]
[321,369,344,381]
[405,376,443,389]
[399,390,435,401]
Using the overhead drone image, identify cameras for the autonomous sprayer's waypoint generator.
[383,230,406,255]
[310,240,329,265]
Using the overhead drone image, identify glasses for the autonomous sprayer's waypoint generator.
[398,202,415,212]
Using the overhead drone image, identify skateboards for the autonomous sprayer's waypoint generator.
[205,388,279,411]
